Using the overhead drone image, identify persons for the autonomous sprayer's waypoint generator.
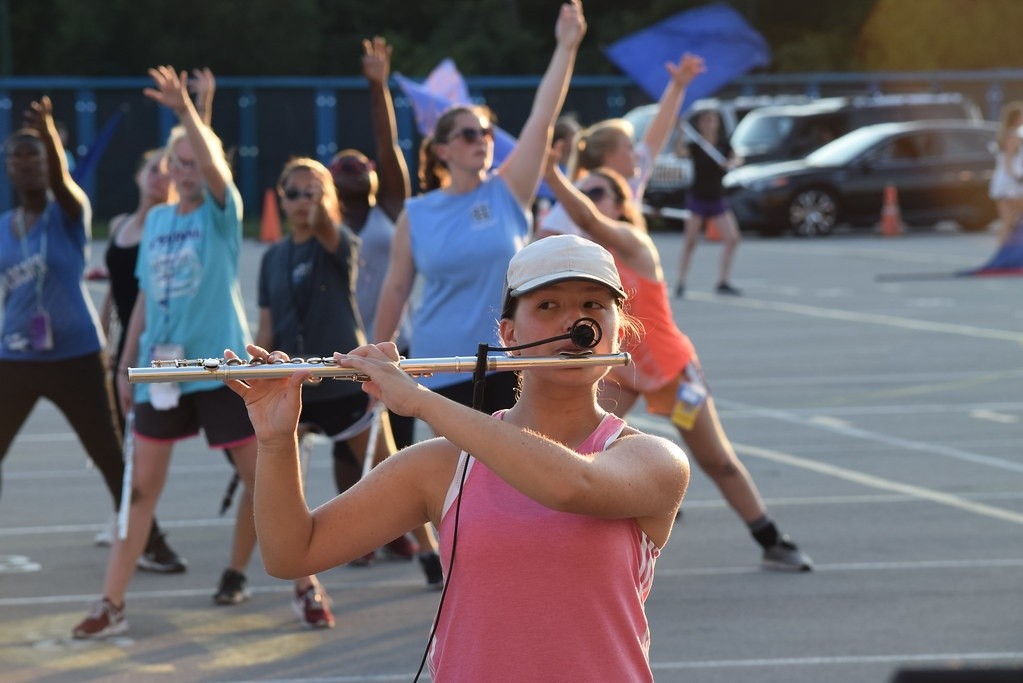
[368,0,589,417]
[72,35,447,637]
[217,234,690,683]
[538,138,813,572]
[523,47,706,253]
[0,99,192,574]
[986,102,1023,247]
[670,101,743,296]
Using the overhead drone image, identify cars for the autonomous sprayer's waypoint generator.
[720,120,1002,237]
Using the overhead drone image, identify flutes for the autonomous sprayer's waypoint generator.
[126,355,639,388]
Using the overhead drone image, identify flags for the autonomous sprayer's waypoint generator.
[399,57,568,200]
[606,1,776,115]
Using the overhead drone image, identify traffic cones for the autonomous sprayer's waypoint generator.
[260,188,283,241]
[877,185,907,236]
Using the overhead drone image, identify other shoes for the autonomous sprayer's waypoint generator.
[350,552,377,567]
[675,277,686,297]
[92,526,113,548]
[714,282,737,295]
[380,533,420,558]
[411,551,446,590]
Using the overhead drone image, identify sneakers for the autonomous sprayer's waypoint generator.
[213,568,252,605]
[290,581,335,629]
[762,538,815,572]
[135,520,188,572]
[71,594,129,640]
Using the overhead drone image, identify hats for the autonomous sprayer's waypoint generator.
[502,234,628,323]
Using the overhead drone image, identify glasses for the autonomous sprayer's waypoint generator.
[446,127,494,150]
[279,183,318,202]
[581,186,619,203]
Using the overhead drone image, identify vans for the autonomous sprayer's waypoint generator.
[727,91,985,175]
[620,94,810,226]
